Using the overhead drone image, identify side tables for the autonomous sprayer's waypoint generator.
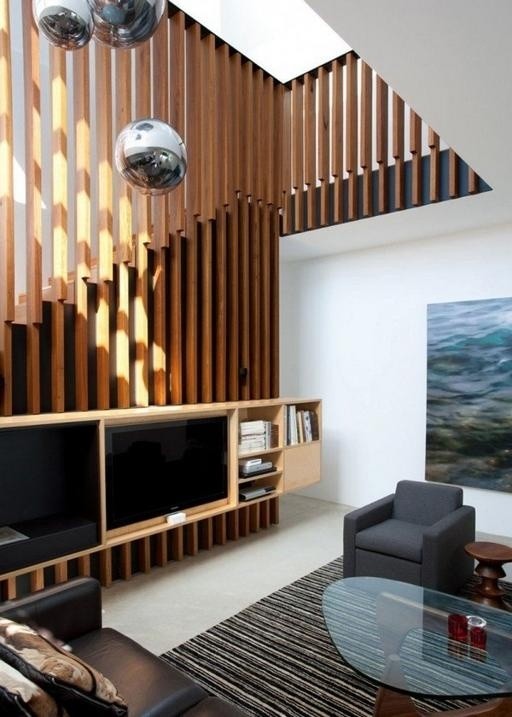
[463,541,511,617]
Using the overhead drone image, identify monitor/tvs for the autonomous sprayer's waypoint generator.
[105,415,228,532]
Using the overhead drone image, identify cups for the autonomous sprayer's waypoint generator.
[447,612,469,642]
[468,616,487,648]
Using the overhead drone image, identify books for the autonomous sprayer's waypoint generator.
[286,405,319,447]
[237,416,274,456]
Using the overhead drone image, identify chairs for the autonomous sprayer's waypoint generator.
[342,479,476,605]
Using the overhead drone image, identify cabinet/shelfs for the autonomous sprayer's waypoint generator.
[237,398,325,507]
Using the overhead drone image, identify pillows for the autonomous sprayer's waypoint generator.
[2,617,128,714]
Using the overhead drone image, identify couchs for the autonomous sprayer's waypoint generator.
[1,576,253,715]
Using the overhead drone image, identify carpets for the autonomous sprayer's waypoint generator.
[159,557,512,715]
